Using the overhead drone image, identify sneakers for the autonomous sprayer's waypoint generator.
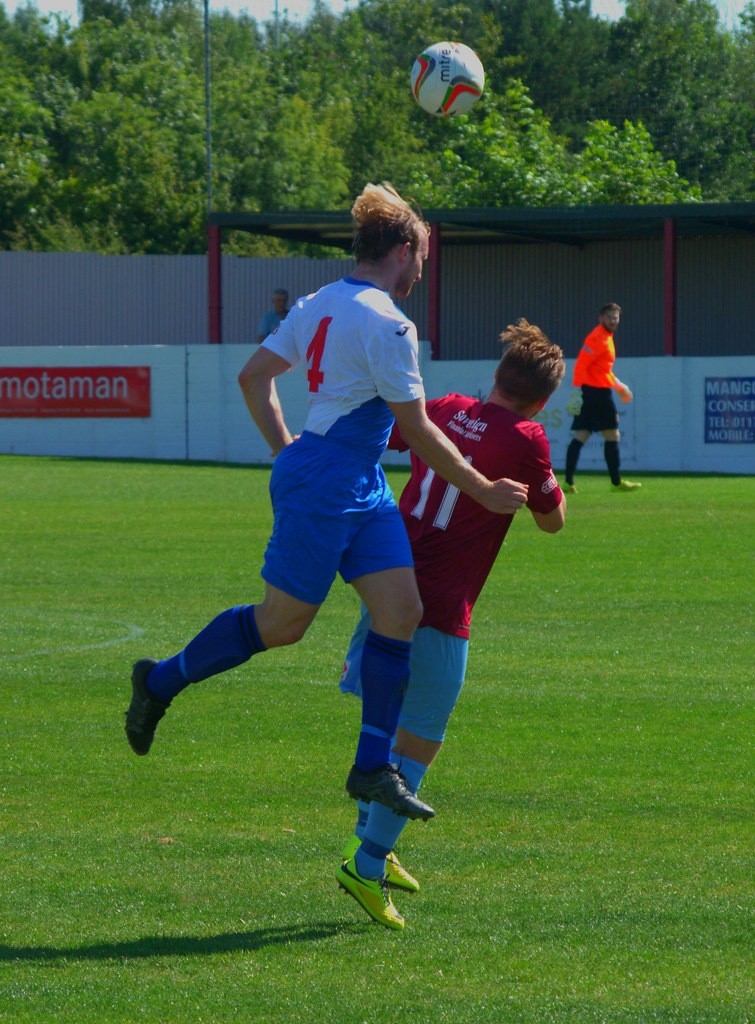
[345,755,436,822]
[123,659,171,756]
[341,833,419,894]
[611,479,641,491]
[334,859,405,931]
[562,483,578,495]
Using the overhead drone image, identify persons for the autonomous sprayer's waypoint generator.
[123,184,529,822]
[257,288,289,341]
[559,302,642,495]
[333,317,567,929]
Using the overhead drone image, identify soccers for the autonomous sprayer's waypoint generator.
[410,40,486,120]
